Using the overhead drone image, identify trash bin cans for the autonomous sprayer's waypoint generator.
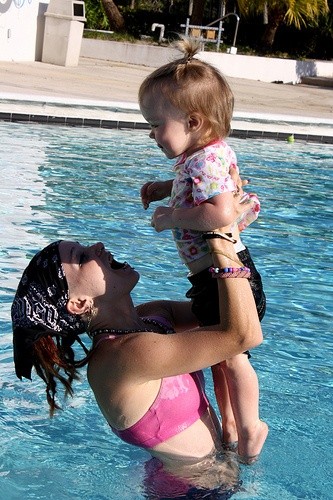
[41,0,87,67]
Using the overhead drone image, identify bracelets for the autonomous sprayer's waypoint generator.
[203,230,251,279]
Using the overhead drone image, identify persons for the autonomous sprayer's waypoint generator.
[137,31,269,467]
[9,165,264,500]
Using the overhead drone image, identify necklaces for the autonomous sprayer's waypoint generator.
[90,317,175,335]
